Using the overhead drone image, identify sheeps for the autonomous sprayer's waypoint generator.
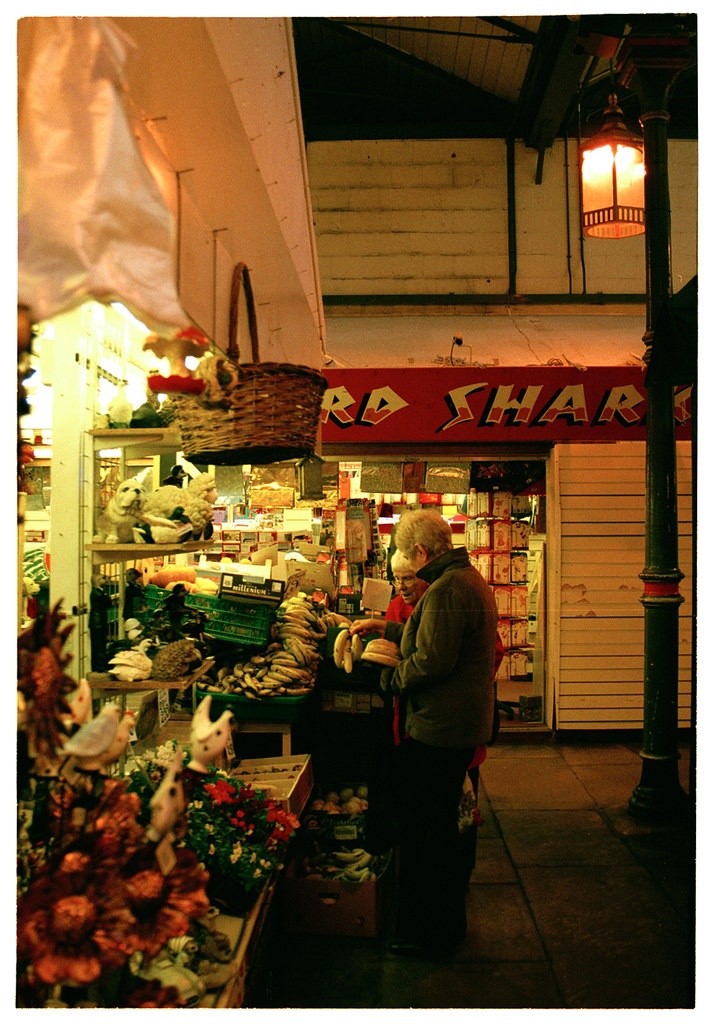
[140,471,218,542]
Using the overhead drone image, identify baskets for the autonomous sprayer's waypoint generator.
[170,259,328,464]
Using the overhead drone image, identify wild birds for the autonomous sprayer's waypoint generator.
[187,695,239,776]
[140,748,189,849]
[56,676,138,774]
[108,638,158,682]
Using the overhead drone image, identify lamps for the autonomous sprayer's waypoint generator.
[580,58,646,239]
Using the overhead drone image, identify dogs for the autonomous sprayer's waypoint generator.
[91,478,147,544]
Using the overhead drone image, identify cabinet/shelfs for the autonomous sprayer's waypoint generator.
[155,719,294,772]
[87,428,215,567]
[83,659,217,725]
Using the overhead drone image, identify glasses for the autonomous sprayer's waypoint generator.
[390,576,418,588]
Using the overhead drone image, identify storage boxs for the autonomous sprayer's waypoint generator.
[105,580,276,650]
[200,689,307,723]
[281,844,396,939]
[169,260,329,469]
[228,753,314,818]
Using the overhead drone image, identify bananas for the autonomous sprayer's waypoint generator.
[196,591,402,700]
[303,849,375,904]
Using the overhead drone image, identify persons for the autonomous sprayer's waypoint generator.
[347,510,500,963]
[380,548,507,881]
[123,569,146,622]
[164,584,198,637]
[89,574,114,672]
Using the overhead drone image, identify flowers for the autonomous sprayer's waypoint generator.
[126,738,301,900]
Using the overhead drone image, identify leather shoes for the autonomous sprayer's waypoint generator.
[390,936,461,958]
